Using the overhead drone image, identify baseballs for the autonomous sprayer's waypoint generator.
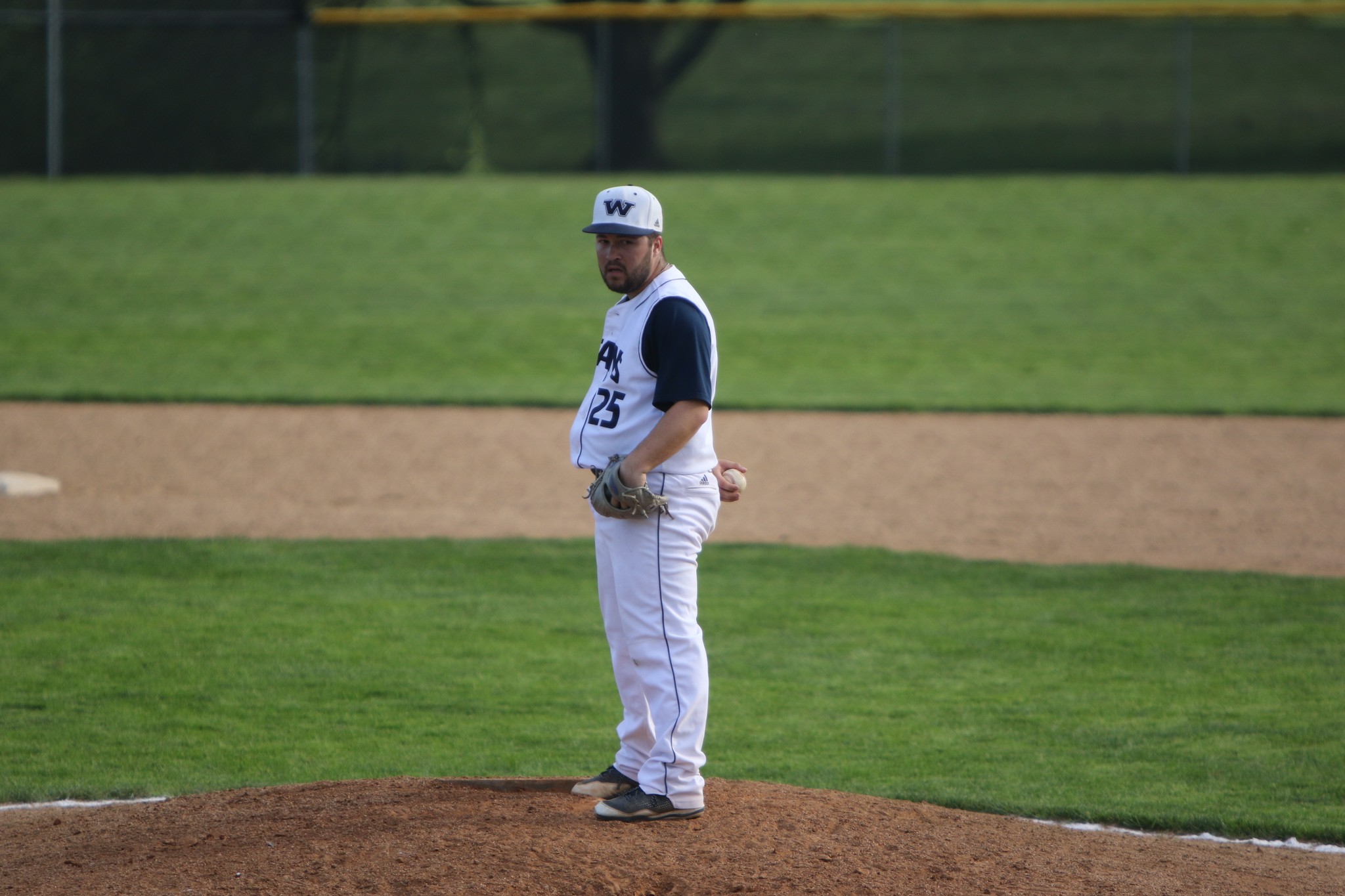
[721,469,747,493]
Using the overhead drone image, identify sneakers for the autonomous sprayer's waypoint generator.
[571,766,640,799]
[593,784,705,820]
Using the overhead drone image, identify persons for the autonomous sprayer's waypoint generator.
[569,184,747,818]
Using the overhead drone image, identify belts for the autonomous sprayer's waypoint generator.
[591,467,605,478]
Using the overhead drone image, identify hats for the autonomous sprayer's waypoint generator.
[582,183,663,236]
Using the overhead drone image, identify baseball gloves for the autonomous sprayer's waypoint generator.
[590,453,660,520]
